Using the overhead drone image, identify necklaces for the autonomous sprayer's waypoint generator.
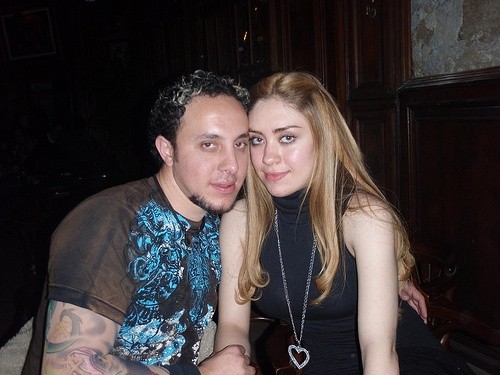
[275,205,318,369]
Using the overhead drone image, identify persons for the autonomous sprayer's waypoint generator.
[20,70,257,375]
[214,73,469,373]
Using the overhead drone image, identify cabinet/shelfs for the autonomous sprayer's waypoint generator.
[395,67,500,375]
